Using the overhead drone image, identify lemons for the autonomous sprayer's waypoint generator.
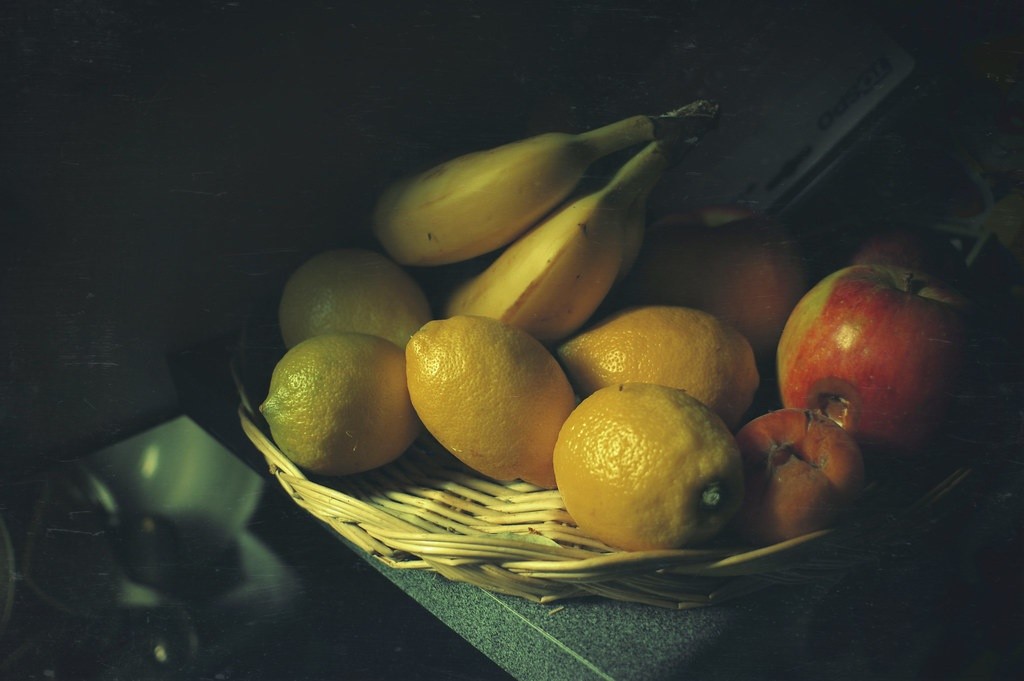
[279,247,430,352]
[258,333,419,475]
[553,384,744,548]
[406,316,576,488]
[554,305,758,424]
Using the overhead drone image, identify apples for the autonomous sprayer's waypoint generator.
[777,264,969,454]
[634,208,806,352]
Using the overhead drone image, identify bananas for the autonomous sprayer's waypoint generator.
[370,100,718,348]
